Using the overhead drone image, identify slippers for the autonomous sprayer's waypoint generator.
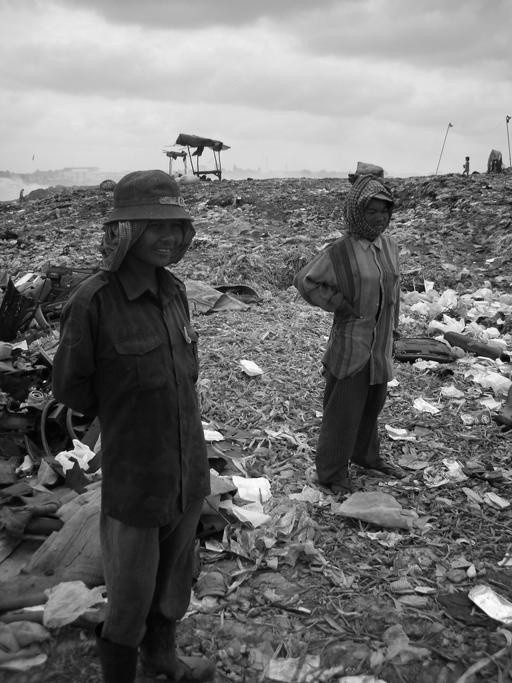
[379,461,406,478]
[331,477,357,496]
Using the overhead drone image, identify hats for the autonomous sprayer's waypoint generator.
[102,171,195,225]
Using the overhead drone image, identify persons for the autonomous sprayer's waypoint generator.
[462,156,469,177]
[293,172,407,502]
[51,170,218,683]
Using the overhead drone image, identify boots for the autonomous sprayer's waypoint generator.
[140,629,216,681]
[96,620,139,683]
